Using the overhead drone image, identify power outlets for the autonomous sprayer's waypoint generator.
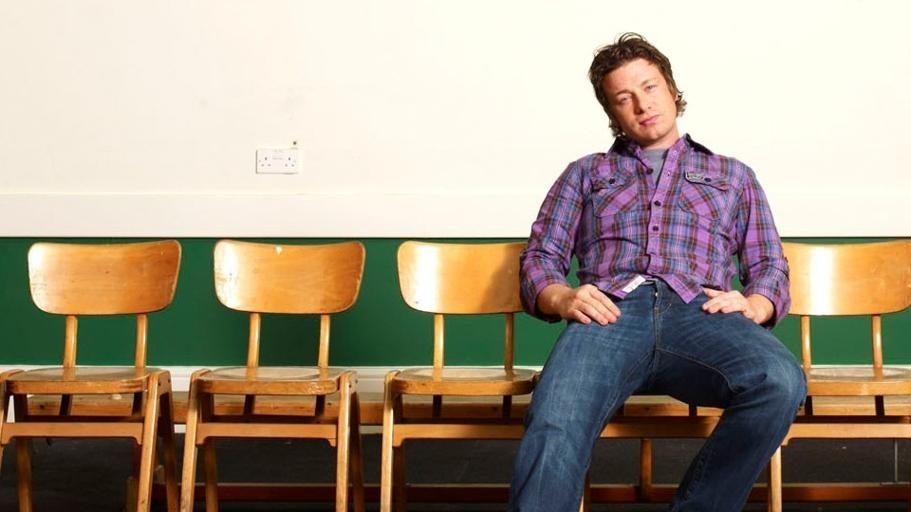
[255,148,300,174]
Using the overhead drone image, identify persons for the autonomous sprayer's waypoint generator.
[507,31,811,512]
